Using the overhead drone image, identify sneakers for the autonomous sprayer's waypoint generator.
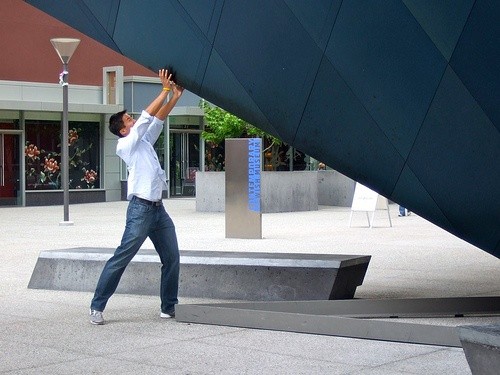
[160,309,175,318]
[90,308,104,325]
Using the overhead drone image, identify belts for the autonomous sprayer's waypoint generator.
[132,196,163,207]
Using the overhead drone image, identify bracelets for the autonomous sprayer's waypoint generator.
[162,88,170,91]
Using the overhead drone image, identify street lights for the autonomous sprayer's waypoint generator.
[51,38,81,221]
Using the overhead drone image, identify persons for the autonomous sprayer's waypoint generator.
[88,69,184,326]
[397,205,411,217]
